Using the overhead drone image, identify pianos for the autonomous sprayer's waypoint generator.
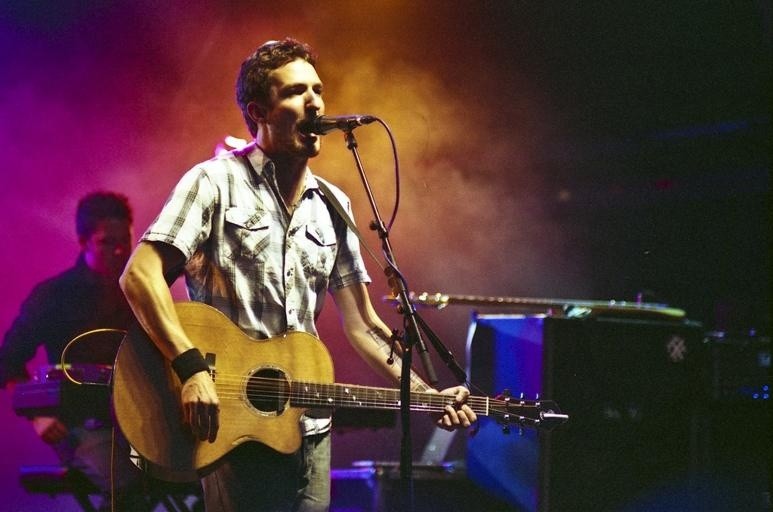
[12,362,114,416]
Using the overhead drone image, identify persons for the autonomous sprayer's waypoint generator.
[0,188,162,512]
[118,36,479,510]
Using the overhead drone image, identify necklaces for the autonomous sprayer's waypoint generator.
[275,170,309,209]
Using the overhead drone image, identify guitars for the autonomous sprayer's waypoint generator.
[382,294,686,320]
[108,301,569,484]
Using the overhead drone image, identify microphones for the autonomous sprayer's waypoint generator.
[311,111,377,136]
